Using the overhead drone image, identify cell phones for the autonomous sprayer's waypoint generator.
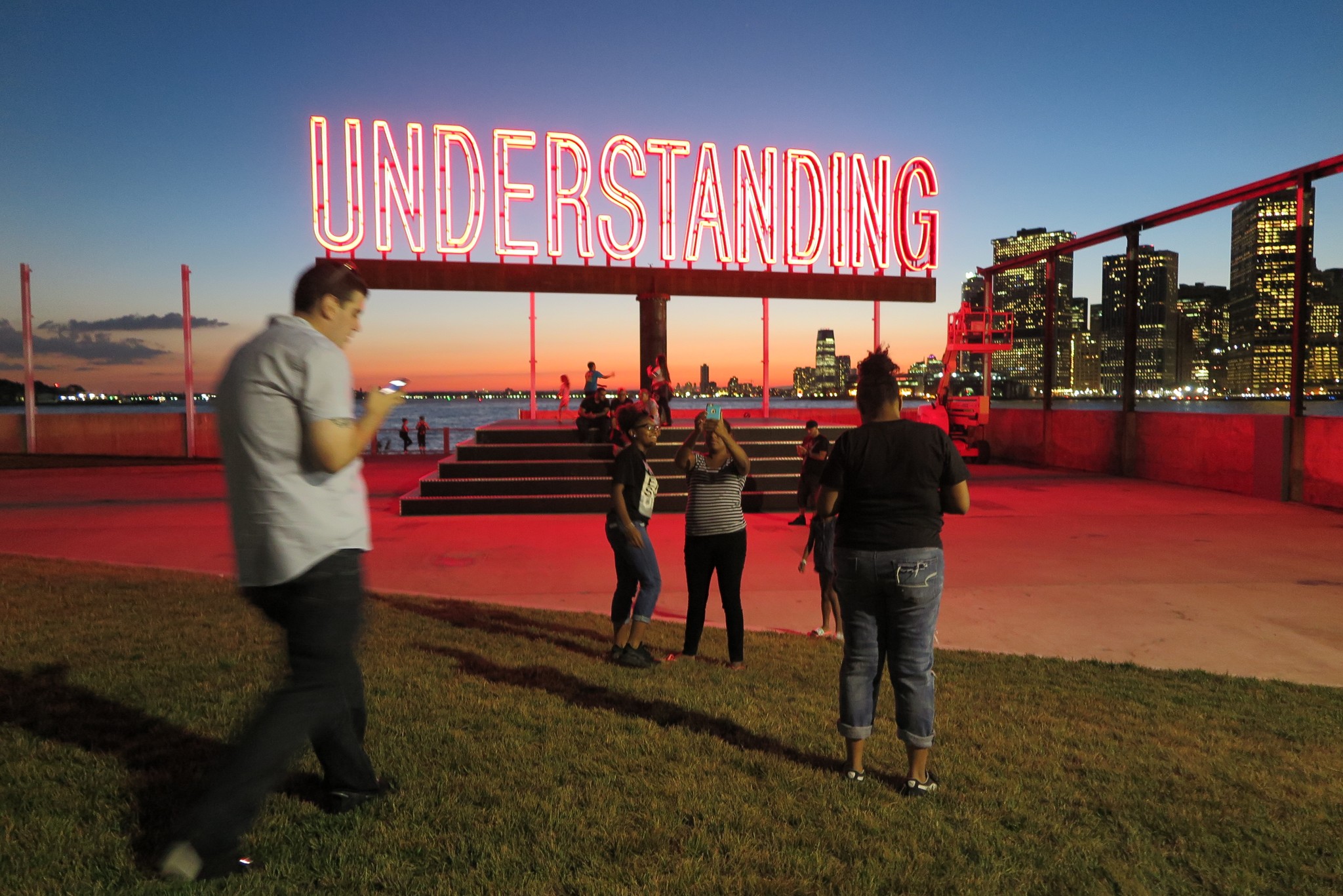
[706,403,721,420]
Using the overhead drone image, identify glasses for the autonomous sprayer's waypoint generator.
[632,424,661,432]
[344,259,359,273]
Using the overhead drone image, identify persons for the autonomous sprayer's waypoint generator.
[659,410,750,674]
[416,415,430,458]
[815,349,971,795]
[605,404,664,669]
[399,417,412,455]
[798,487,845,645]
[788,420,830,526]
[156,262,405,880]
[553,354,673,443]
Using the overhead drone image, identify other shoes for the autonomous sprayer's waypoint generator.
[730,664,745,672]
[663,651,695,662]
[787,515,806,525]
[610,644,623,659]
[322,780,397,813]
[617,641,661,668]
[165,843,264,881]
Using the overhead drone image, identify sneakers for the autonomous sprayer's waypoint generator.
[904,767,939,797]
[843,761,865,781]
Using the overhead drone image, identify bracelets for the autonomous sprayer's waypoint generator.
[801,559,806,567]
[623,523,636,532]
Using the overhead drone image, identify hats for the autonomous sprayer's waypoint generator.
[804,419,818,430]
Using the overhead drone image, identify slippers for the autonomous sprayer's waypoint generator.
[835,633,843,639]
[809,627,831,636]
